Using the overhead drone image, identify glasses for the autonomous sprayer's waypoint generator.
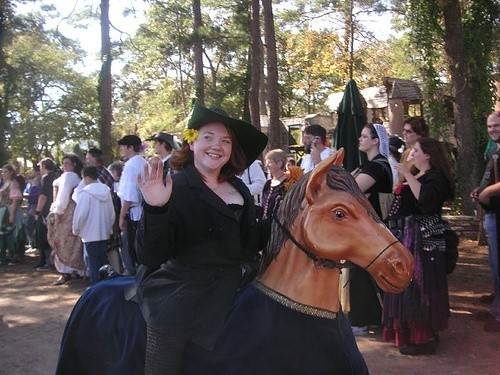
[403,129,414,134]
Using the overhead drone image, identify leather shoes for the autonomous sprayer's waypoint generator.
[53,272,79,286]
[398,333,441,355]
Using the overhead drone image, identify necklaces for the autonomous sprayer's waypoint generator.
[227,190,238,197]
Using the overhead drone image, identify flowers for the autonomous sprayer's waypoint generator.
[183,128,199,143]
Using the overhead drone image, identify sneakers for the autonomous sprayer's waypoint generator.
[473,309,500,331]
[351,325,379,335]
[480,294,495,303]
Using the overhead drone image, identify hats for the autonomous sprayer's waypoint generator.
[187,99,268,175]
[388,137,406,150]
[144,132,176,149]
[118,135,142,145]
[37,157,54,166]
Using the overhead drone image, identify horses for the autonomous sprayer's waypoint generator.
[54,147,416,375]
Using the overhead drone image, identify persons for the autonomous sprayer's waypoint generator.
[236,116,455,355]
[0,109,184,291]
[135,98,260,375]
[469,110,500,332]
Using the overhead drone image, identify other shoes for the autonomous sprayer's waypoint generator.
[8,261,22,266]
[25,248,37,254]
[36,265,52,271]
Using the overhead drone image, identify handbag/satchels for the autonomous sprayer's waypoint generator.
[372,160,395,221]
